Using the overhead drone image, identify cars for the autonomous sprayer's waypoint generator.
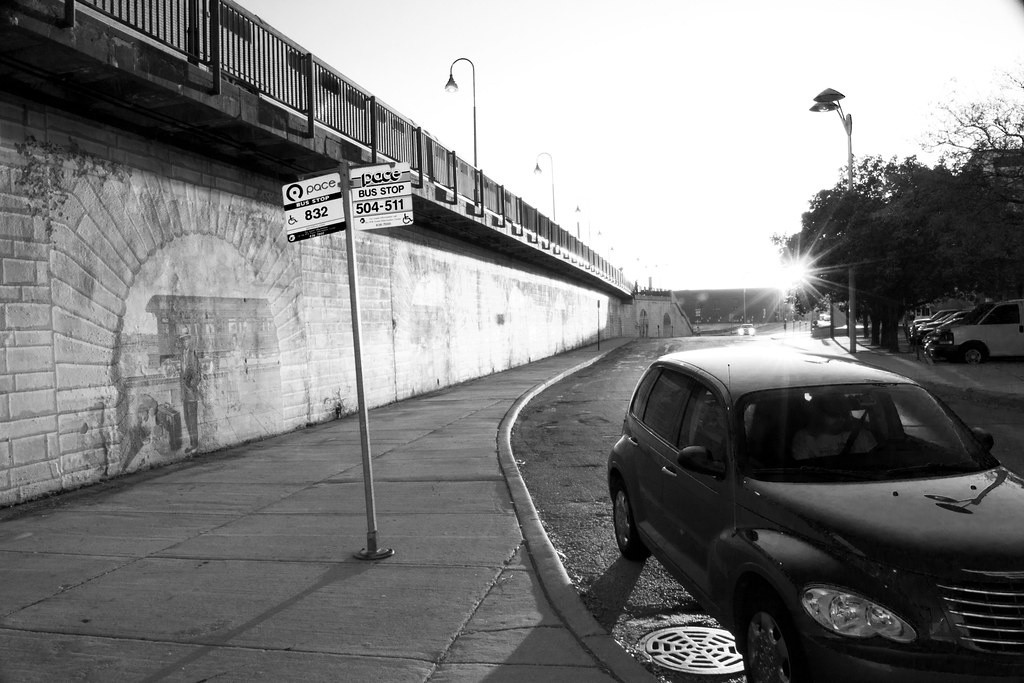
[737,322,755,335]
[910,308,979,355]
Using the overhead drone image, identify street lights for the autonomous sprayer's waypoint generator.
[446,57,479,168]
[805,85,858,356]
[533,150,557,225]
[575,205,626,273]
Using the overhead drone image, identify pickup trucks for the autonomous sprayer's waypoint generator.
[922,296,1024,366]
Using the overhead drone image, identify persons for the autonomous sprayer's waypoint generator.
[792,402,880,466]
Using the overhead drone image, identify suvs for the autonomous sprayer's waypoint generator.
[606,350,1024,682]
